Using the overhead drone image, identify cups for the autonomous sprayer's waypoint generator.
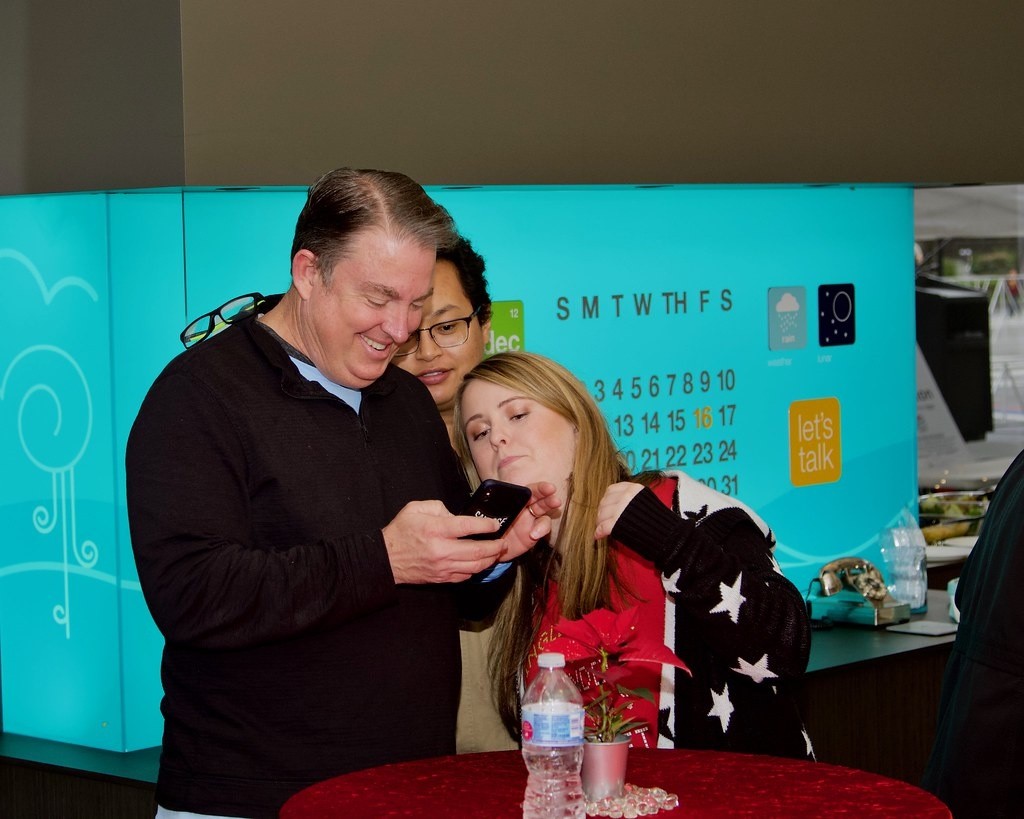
[881,545,929,608]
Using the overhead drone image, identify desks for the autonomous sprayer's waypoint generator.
[288,748,953,819]
[805,586,959,784]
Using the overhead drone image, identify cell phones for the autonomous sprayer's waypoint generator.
[456,479,533,540]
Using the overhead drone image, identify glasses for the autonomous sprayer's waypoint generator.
[179,292,266,348]
[394,306,483,356]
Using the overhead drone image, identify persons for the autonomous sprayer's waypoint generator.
[125,168,562,818]
[450,352,820,762]
[927,441,1024,819]
[386,233,520,753]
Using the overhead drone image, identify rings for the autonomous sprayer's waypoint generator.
[529,506,539,517]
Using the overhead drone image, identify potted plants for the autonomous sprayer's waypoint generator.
[547,604,694,800]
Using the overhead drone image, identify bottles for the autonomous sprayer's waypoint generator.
[521,652,590,815]
